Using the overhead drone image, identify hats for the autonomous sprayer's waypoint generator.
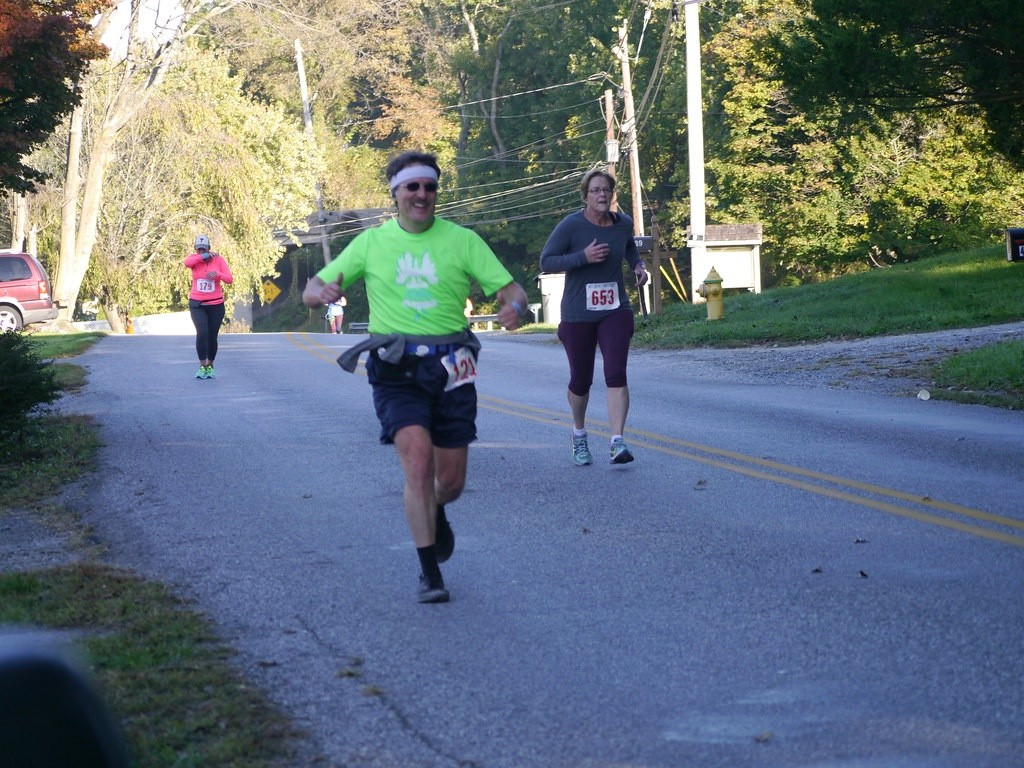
[195,235,209,250]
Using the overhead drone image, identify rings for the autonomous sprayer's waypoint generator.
[211,274,212,277]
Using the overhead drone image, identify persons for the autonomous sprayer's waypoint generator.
[324,296,346,335]
[184,235,233,379]
[540,171,648,467]
[301,153,529,603]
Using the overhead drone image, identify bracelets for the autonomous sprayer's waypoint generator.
[511,301,524,318]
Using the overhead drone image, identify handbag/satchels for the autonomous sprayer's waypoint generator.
[189,299,201,308]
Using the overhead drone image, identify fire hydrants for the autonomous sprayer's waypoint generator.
[695,265,725,321]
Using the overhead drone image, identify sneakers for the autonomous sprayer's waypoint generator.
[572,431,593,466]
[205,365,216,378]
[417,568,449,603]
[608,438,634,464]
[195,365,207,379]
[434,504,454,563]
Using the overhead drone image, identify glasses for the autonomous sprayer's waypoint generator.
[587,187,613,194]
[400,182,438,192]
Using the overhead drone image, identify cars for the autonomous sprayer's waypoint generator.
[0,253,59,334]
